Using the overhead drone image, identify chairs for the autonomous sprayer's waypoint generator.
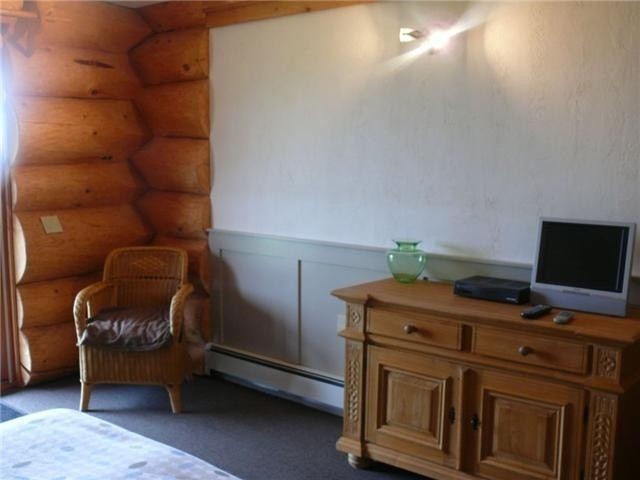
[72,246,196,413]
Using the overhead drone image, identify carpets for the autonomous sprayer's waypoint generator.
[1,405,239,479]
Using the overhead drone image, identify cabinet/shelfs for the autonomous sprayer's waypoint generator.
[329,277,639,479]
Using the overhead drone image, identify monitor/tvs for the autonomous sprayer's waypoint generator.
[528,216,636,317]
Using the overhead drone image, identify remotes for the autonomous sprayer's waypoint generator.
[520,304,552,319]
[553,310,575,324]
